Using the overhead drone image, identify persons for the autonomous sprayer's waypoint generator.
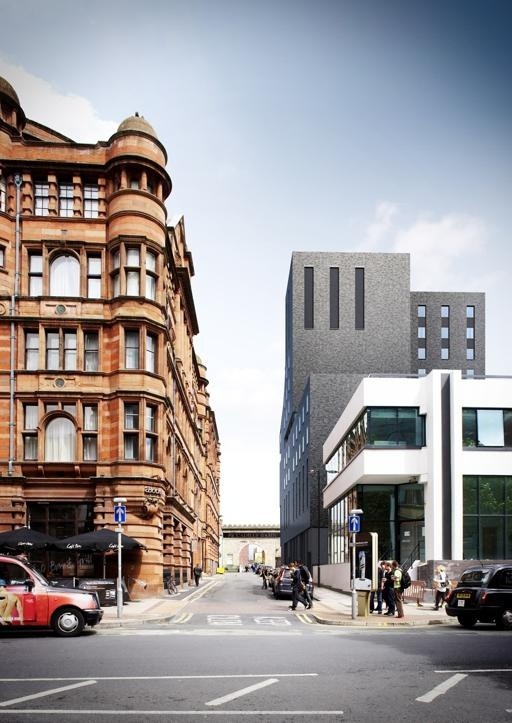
[293,560,313,610]
[194,563,203,586]
[0,579,24,626]
[261,566,269,589]
[431,565,449,610]
[366,560,405,618]
[287,562,310,611]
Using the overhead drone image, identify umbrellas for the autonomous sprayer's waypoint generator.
[0,526,62,561]
[51,528,147,579]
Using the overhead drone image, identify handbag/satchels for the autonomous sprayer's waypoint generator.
[439,575,450,588]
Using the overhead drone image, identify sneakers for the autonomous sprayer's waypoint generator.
[432,605,443,610]
[289,605,297,610]
[369,608,404,618]
[305,603,312,609]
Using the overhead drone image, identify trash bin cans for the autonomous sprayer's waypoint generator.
[351,578,371,617]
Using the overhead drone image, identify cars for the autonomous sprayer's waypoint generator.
[0,554,103,637]
[445,563,512,630]
[255,564,314,600]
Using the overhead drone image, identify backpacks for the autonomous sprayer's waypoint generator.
[394,568,412,589]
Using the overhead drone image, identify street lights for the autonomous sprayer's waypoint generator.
[308,469,321,587]
[218,514,223,568]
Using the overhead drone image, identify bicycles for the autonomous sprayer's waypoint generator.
[167,576,178,594]
